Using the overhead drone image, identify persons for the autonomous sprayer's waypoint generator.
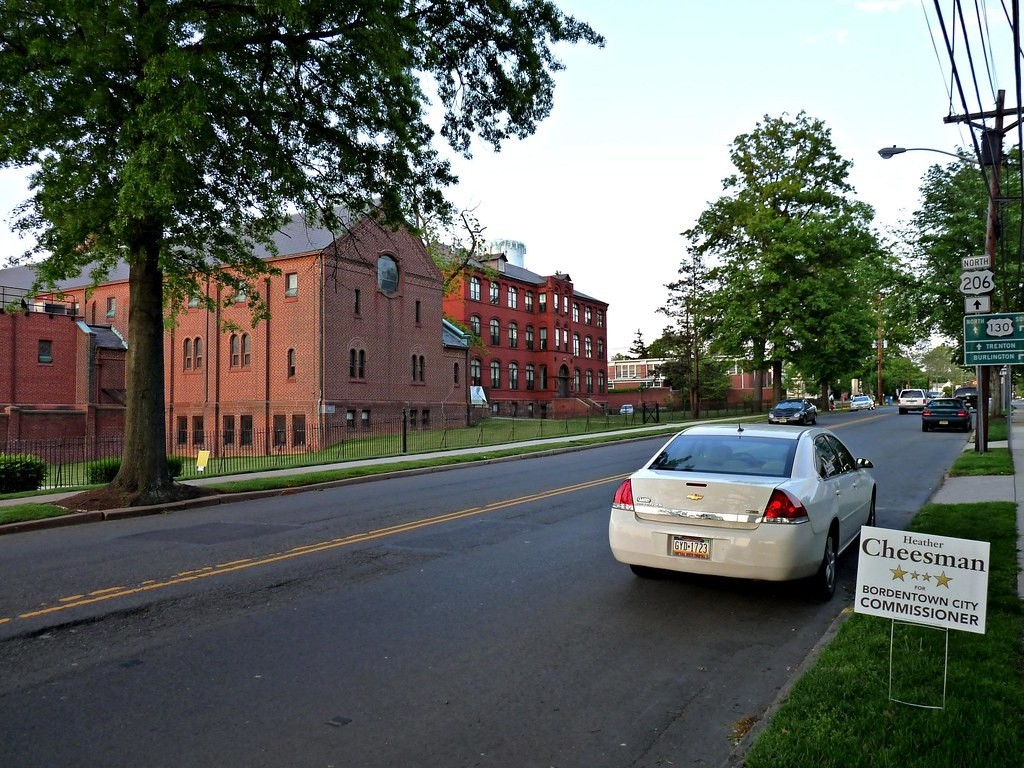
[871,393,876,406]
[829,392,835,405]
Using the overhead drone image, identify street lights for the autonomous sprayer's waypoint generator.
[877,144,1002,455]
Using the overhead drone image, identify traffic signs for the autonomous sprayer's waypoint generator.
[965,313,1024,366]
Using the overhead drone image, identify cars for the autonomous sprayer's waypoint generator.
[620,404,633,415]
[929,392,942,398]
[607,424,877,601]
[768,399,817,426]
[921,397,973,433]
[850,396,874,411]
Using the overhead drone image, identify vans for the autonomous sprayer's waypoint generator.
[898,388,928,415]
[952,386,977,410]
